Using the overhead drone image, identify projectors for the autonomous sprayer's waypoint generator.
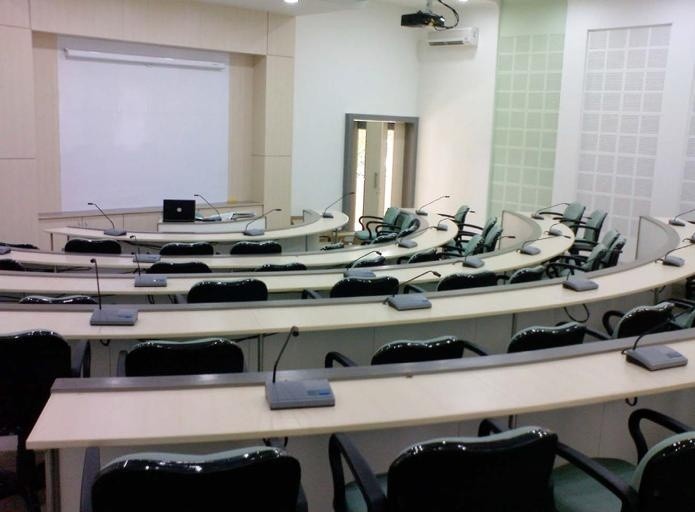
[401,10,446,31]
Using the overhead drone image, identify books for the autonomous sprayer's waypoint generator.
[231,213,254,221]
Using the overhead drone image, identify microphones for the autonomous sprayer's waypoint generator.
[129,251,167,287]
[88,202,127,236]
[130,235,161,263]
[242,208,282,236]
[562,249,623,292]
[321,191,593,280]
[194,194,222,222]
[264,326,336,411]
[624,307,695,372]
[662,208,695,267]
[387,269,442,310]
[89,259,139,326]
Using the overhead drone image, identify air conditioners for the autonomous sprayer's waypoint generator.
[426,26,480,46]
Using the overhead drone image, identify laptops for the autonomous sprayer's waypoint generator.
[163,199,196,222]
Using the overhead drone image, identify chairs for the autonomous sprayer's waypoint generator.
[0,204,695,512]
